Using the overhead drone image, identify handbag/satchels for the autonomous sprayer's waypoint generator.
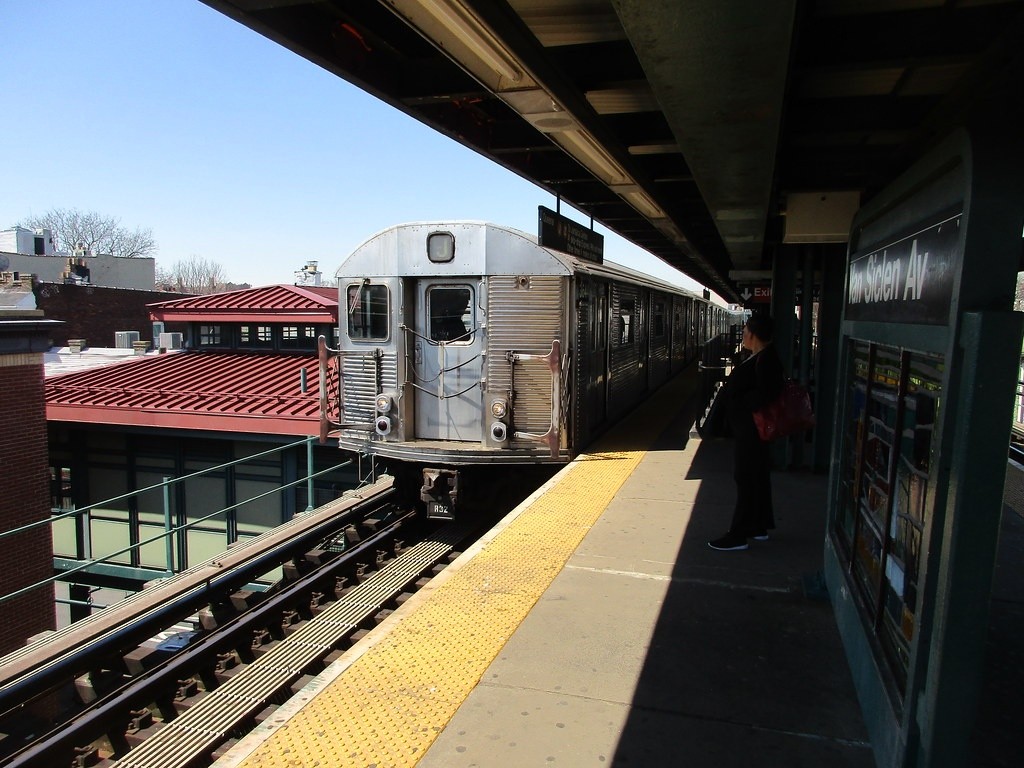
[749,382,816,447]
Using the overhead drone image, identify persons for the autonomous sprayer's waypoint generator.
[707,319,782,550]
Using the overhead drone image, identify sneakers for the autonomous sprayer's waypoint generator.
[728,528,769,542]
[708,532,749,551]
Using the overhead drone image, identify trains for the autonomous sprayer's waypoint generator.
[318,219,752,523]
